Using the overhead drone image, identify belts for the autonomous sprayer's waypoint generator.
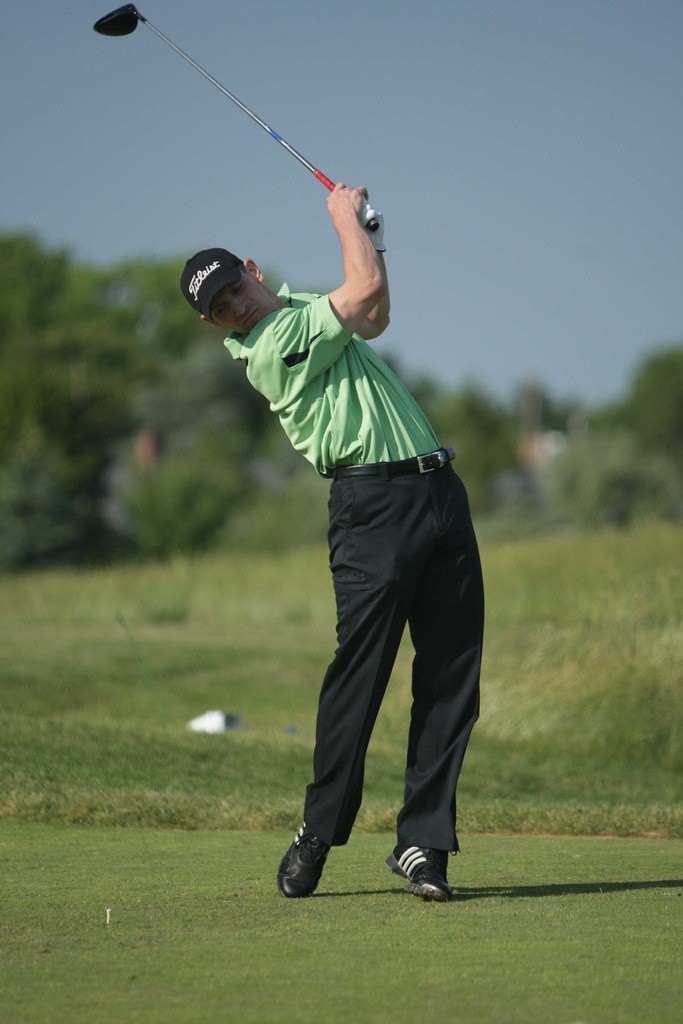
[335,447,456,480]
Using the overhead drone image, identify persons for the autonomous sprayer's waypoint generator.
[180,184,485,897]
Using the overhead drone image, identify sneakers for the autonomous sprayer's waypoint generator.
[385,844,458,901]
[276,820,330,897]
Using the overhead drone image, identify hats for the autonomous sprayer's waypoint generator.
[181,248,244,320]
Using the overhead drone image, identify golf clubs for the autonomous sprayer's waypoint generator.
[92,4,381,234]
